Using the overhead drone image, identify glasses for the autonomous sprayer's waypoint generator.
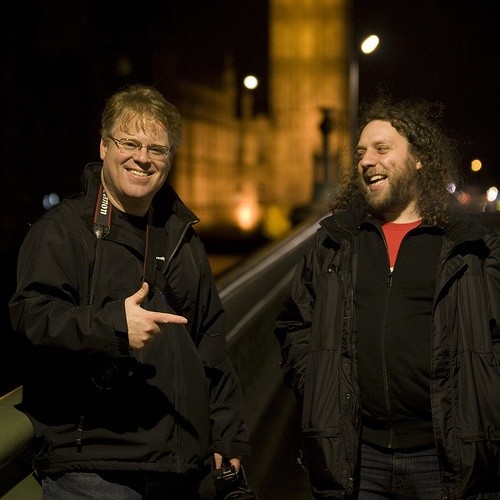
[107,133,172,160]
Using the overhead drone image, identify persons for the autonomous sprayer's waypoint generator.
[8,86,252,500]
[272,101,500,500]
